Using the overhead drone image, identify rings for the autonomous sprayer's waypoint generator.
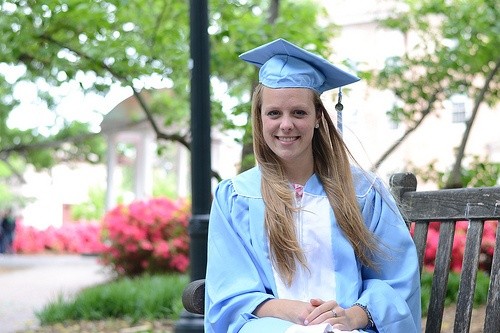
[332,309,337,317]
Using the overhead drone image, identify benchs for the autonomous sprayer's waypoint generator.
[182,171,500,333]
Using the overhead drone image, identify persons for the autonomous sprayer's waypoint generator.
[204,39,422,333]
[0,208,16,254]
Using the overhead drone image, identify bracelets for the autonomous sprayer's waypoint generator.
[353,303,374,331]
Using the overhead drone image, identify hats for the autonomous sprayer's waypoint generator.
[238,37,360,139]
